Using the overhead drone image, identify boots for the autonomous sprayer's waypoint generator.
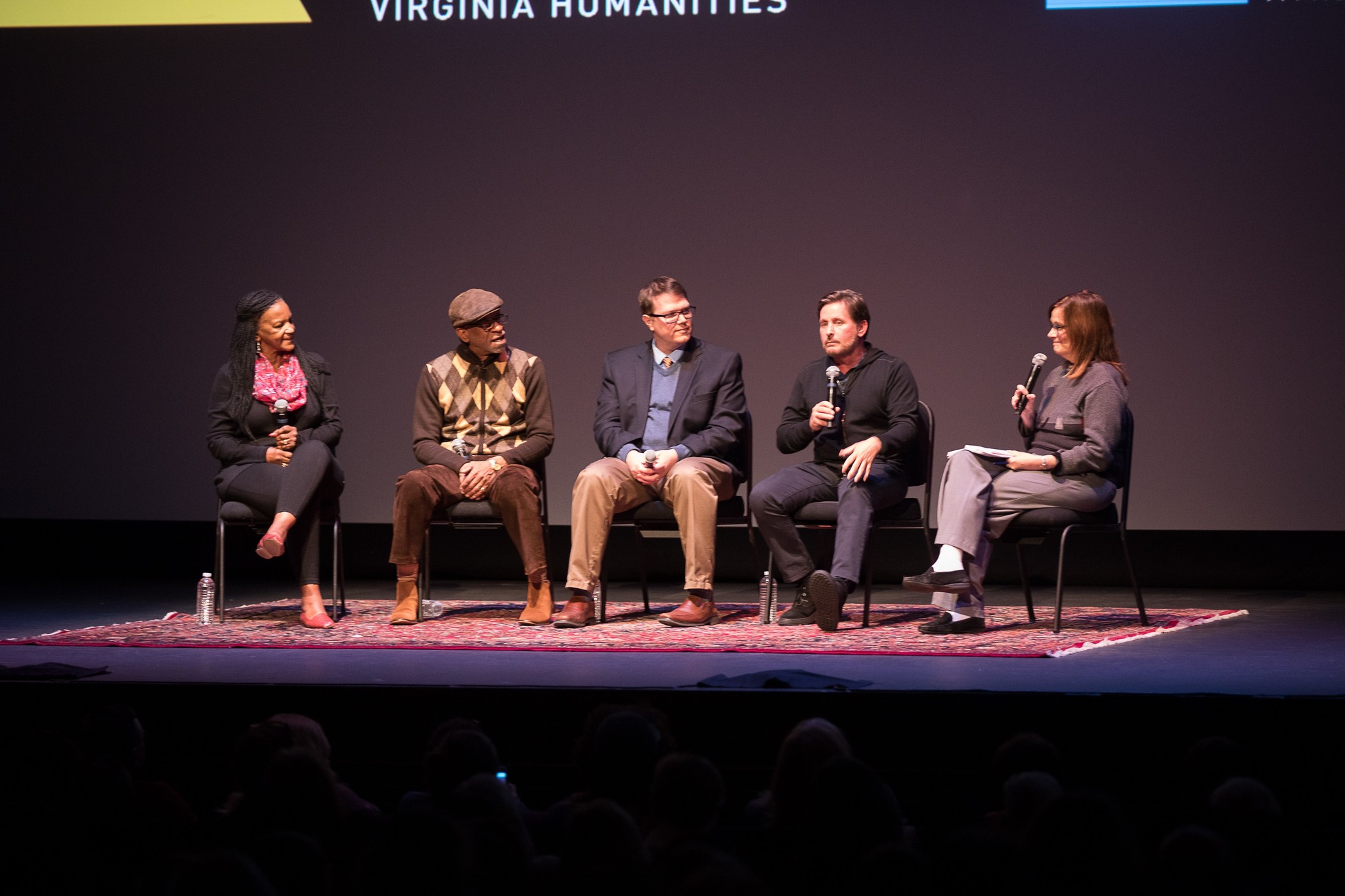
[517,581,554,625]
[389,581,419,624]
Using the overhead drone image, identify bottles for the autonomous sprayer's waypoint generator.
[592,580,602,623]
[419,599,443,617]
[759,571,778,624]
[196,573,215,626]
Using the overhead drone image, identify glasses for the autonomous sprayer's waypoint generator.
[648,305,696,324]
[1050,323,1069,331]
[468,314,509,330]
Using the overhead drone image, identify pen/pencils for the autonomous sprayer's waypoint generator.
[993,462,1008,464]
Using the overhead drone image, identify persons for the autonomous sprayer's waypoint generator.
[749,289,920,632]
[0,701,1287,896]
[390,289,554,625]
[554,277,746,628]
[206,291,346,630]
[903,291,1128,634]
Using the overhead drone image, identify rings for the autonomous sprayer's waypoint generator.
[281,463,286,467]
[656,479,659,480]
[481,488,487,491]
[284,439,288,444]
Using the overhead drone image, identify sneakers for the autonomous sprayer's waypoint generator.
[778,585,817,625]
[903,566,971,594]
[918,611,986,633]
[808,569,848,630]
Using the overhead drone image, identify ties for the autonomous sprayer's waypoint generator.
[662,357,672,369]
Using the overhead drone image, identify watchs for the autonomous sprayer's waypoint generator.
[1041,455,1047,470]
[486,458,501,472]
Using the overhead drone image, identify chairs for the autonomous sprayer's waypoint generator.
[595,415,759,621]
[418,456,555,625]
[213,449,346,624]
[988,407,1148,633]
[764,399,935,628]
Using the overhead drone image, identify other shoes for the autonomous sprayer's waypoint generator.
[300,612,335,628]
[255,534,285,559]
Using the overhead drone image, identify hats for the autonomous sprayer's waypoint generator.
[448,289,504,328]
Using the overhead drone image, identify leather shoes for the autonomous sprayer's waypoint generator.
[659,591,719,626]
[553,595,598,626]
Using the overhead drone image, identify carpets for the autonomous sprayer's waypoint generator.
[0,598,1248,660]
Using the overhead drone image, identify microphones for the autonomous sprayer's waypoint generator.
[644,449,655,468]
[452,438,470,460]
[1015,353,1048,414]
[826,366,839,428]
[274,399,288,428]
